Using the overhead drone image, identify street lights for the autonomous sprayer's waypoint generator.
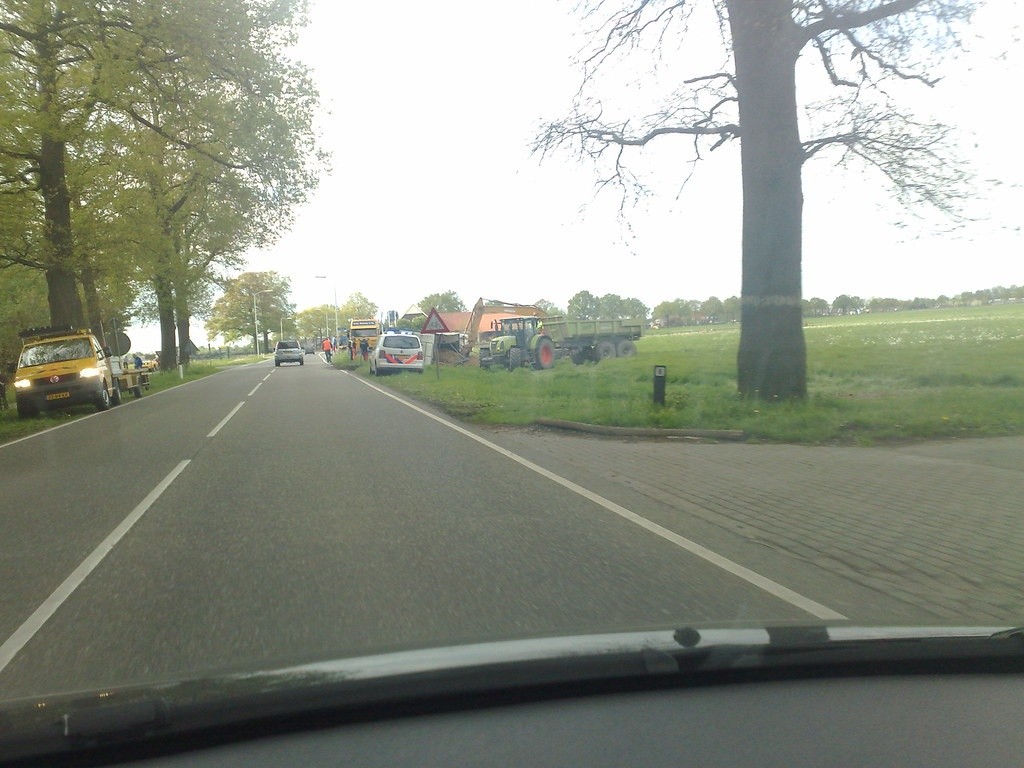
[252,290,274,355]
[280,317,293,342]
[315,275,338,349]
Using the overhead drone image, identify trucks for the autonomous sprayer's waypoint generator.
[349,317,383,353]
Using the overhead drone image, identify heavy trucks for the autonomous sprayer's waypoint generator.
[14,324,150,419]
[479,315,644,371]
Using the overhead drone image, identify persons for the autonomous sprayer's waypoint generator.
[360,338,370,362]
[0,376,10,410]
[123,356,128,369]
[348,337,356,360]
[322,336,338,364]
[132,354,142,369]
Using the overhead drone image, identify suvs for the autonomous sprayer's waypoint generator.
[366,332,424,376]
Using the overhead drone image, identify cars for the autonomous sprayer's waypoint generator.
[274,339,304,366]
[305,342,315,354]
